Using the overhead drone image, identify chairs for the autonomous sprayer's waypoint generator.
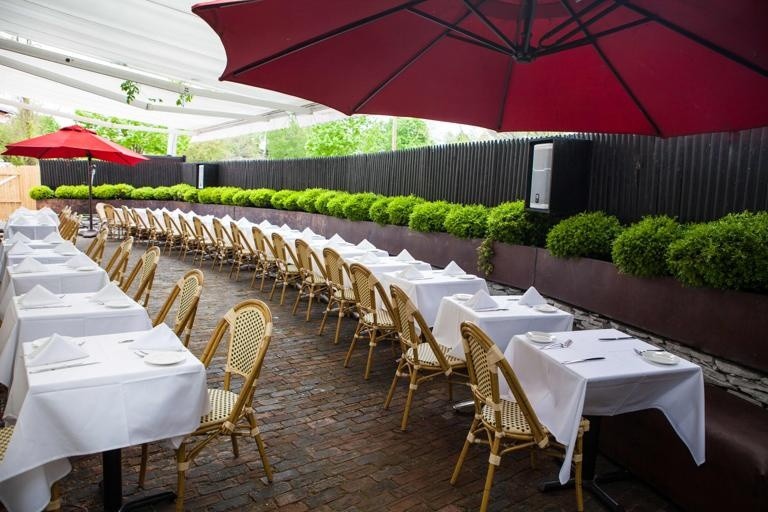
[343,262,405,380]
[192,216,218,268]
[84,228,108,267]
[382,283,469,432]
[451,321,589,512]
[148,268,205,348]
[269,235,302,306]
[55,203,82,247]
[251,226,280,288]
[104,204,124,241]
[136,298,272,491]
[162,212,190,256]
[120,205,140,243]
[105,236,135,285]
[177,214,208,262]
[122,247,160,312]
[130,208,150,248]
[291,238,335,321]
[211,218,235,272]
[229,222,258,282]
[145,208,172,249]
[317,249,358,344]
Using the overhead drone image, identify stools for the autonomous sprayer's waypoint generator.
[1,427,63,512]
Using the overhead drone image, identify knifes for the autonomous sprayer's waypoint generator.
[567,356,605,364]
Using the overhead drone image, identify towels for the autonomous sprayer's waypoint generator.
[155,207,161,212]
[12,256,48,272]
[518,285,548,304]
[36,212,54,222]
[90,282,131,301]
[278,224,292,231]
[160,207,170,212]
[13,213,29,224]
[27,332,90,367]
[329,233,346,243]
[19,284,63,306]
[63,251,99,269]
[41,230,65,244]
[442,260,467,275]
[359,249,379,263]
[7,229,32,244]
[237,216,250,224]
[187,210,197,215]
[52,240,75,251]
[399,264,424,279]
[173,207,184,213]
[6,240,34,254]
[127,321,187,352]
[258,219,272,226]
[302,227,316,236]
[355,238,376,250]
[464,288,498,309]
[395,248,415,261]
[221,214,234,221]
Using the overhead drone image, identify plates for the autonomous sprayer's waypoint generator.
[536,305,557,311]
[454,294,473,300]
[642,352,679,365]
[107,302,130,308]
[144,352,185,365]
[526,331,555,343]
[78,266,95,271]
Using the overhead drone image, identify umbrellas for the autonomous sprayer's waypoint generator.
[191,0,768,137]
[0,126,151,229]
